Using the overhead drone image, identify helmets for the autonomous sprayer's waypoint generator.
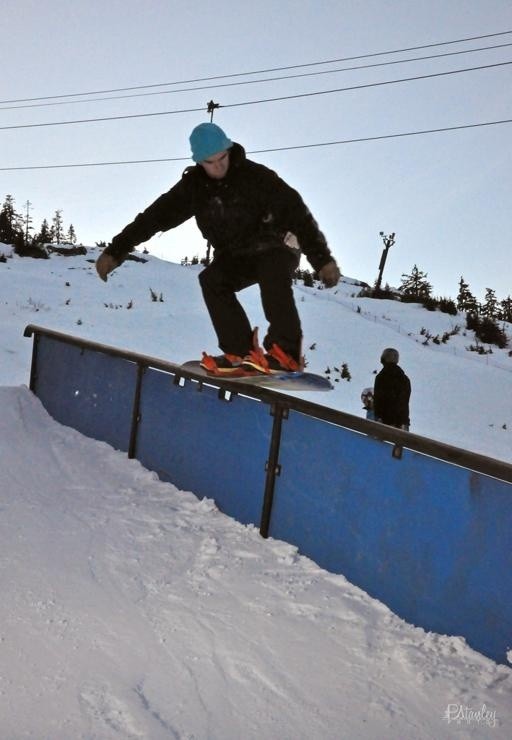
[361,389,374,408]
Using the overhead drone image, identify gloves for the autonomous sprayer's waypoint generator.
[318,261,339,288]
[97,253,119,281]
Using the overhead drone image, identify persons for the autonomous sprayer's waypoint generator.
[362,346,411,429]
[94,123,342,378]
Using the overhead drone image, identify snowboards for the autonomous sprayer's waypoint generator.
[178,361,334,390]
[361,388,374,420]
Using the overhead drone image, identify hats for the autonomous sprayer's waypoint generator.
[190,123,233,162]
[381,349,398,364]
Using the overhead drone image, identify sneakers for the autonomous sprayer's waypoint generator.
[200,352,255,373]
[240,354,304,375]
